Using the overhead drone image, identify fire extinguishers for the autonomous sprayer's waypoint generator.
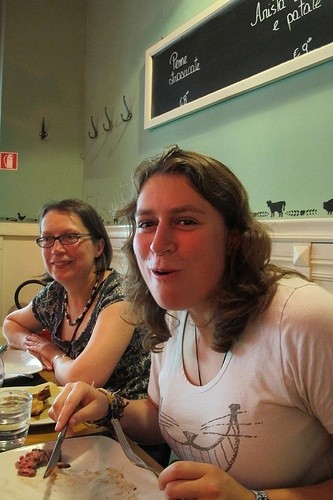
[15,273,54,342]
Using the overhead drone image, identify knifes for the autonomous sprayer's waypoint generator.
[43,424,69,480]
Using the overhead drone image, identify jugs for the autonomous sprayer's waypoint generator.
[0,345,7,388]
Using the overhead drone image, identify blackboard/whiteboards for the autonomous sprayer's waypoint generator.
[143,0,333,131]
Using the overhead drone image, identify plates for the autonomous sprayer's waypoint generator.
[0,435,169,500]
[0,350,44,380]
[0,386,64,424]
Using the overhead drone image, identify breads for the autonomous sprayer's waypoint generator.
[26,381,61,415]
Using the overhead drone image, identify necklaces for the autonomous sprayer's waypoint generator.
[64,272,99,326]
[194,323,229,386]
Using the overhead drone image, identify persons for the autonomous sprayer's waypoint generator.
[3,200,172,467]
[49,145,333,500]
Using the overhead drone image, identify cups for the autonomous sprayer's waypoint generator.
[0,391,32,452]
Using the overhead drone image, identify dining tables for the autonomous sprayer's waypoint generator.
[23,369,164,477]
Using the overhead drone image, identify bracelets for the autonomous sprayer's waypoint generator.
[86,388,130,428]
[52,353,67,365]
[251,490,268,500]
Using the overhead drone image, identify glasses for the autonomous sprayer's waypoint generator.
[36,231,91,248]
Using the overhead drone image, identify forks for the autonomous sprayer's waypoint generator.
[112,418,161,478]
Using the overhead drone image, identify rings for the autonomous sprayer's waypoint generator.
[35,342,40,346]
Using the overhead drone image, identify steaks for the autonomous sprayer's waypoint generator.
[15,449,71,477]
